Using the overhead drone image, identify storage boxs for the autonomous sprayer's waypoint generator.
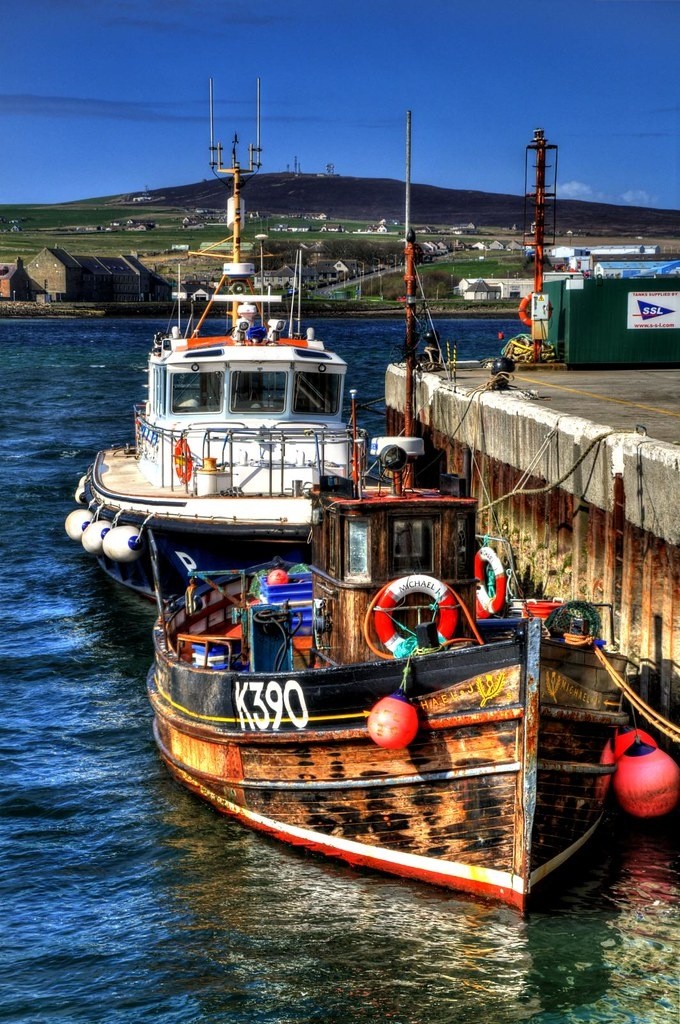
[257,572,313,638]
[192,645,251,673]
[540,276,680,370]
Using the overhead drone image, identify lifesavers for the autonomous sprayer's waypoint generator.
[518,293,553,327]
[175,439,193,485]
[374,574,458,654]
[475,545,507,615]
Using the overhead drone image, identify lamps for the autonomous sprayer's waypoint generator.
[266,318,286,341]
[234,317,251,346]
[306,327,315,341]
[171,326,181,339]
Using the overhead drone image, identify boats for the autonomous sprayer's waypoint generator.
[147,109,680,919]
[65,77,439,619]
